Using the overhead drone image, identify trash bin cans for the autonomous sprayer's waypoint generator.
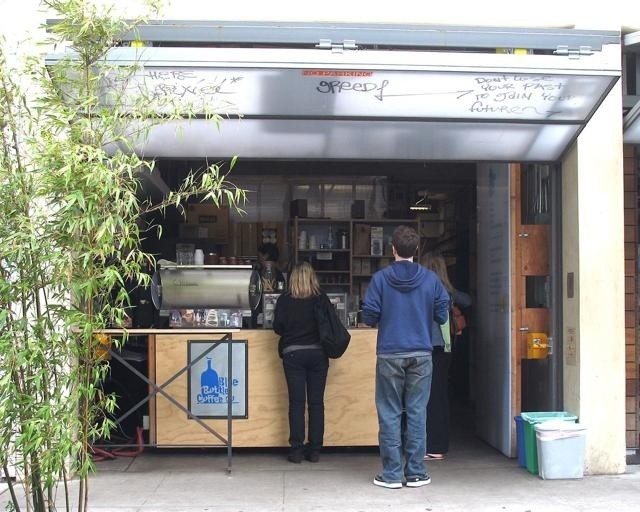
[514,411,587,480]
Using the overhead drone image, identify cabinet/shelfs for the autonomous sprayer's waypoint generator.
[181,162,423,334]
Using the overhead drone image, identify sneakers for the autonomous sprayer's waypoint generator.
[288,455,318,463]
[373,476,402,489]
[406,476,432,487]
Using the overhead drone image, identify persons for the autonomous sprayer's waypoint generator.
[257,243,279,261]
[359,224,450,490]
[273,262,330,463]
[418,253,466,461]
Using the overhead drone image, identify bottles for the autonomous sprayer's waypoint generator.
[327,223,336,248]
[340,232,349,248]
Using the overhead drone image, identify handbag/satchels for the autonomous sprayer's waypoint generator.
[315,293,350,359]
[450,306,466,335]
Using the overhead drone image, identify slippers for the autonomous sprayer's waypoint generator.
[424,454,447,461]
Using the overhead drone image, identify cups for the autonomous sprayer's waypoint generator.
[298,230,317,249]
[175,244,237,264]
